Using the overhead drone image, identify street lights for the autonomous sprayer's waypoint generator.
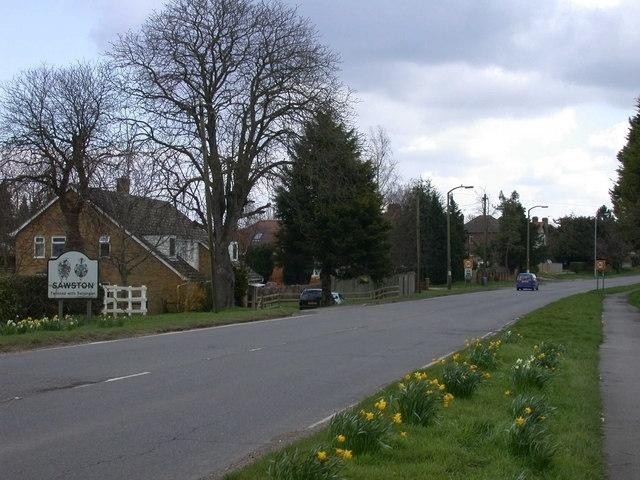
[446,185,475,289]
[594,213,609,277]
[526,205,548,271]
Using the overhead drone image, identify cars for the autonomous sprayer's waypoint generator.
[331,293,344,305]
[298,289,338,309]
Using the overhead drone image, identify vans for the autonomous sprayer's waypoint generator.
[516,273,539,291]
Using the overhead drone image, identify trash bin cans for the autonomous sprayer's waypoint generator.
[481,276,488,285]
